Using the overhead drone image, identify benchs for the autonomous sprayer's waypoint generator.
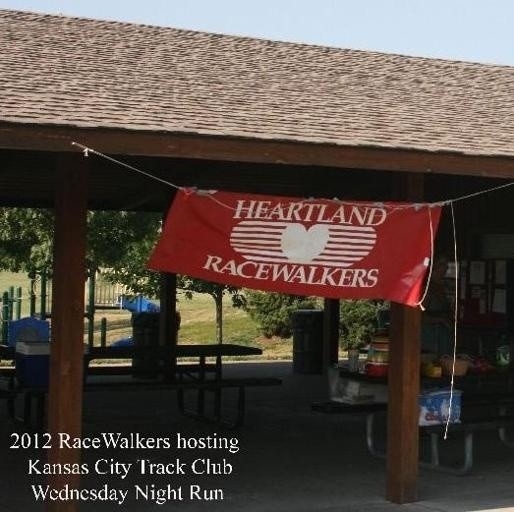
[423,412,514,472]
[310,392,514,456]
[0,376,282,428]
[0,362,218,418]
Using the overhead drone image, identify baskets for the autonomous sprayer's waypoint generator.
[439,354,470,376]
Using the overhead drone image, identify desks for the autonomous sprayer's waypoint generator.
[0,341,262,420]
[339,366,514,465]
